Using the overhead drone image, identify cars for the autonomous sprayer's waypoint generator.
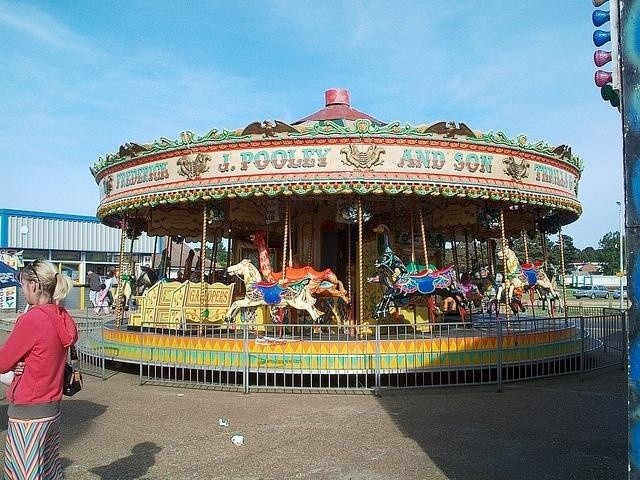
[613,286,628,299]
[559,275,572,286]
[573,285,609,299]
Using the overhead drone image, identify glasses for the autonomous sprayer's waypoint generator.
[28,260,40,282]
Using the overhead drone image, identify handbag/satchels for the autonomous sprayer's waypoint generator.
[63,346,83,396]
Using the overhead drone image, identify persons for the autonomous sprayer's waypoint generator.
[87,270,118,316]
[0,259,79,480]
[442,274,478,312]
